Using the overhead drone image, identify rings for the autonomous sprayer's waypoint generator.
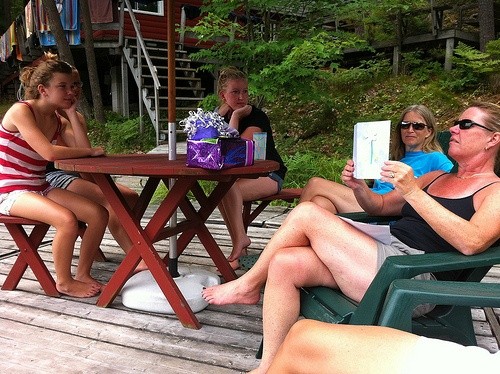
[390,171,395,178]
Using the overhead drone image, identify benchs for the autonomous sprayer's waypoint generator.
[0,214,108,298]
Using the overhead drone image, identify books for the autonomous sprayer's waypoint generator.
[353,120,390,179]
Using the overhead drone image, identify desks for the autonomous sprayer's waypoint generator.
[54,154,280,329]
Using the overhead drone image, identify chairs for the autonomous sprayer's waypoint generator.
[243,131,500,360]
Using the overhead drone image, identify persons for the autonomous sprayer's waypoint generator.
[45,66,165,274]
[267,319,500,374]
[201,101,500,374]
[217,68,287,276]
[0,60,123,298]
[238,105,453,272]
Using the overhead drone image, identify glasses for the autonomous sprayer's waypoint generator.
[454,119,495,136]
[399,121,430,131]
[73,80,83,89]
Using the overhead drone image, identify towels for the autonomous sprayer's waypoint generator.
[0,0,113,62]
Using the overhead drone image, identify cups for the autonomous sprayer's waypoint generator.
[253,132,267,161]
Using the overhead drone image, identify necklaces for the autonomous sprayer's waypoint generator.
[455,173,494,179]
